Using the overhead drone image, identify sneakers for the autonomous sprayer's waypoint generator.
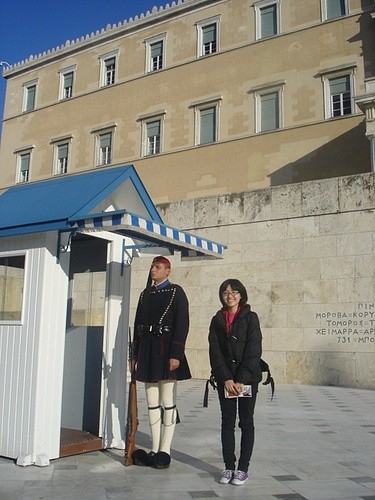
[231,470,249,485]
[220,470,233,483]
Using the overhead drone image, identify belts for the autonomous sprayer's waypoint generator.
[136,322,175,337]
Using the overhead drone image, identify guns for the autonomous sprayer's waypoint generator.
[124,327,139,466]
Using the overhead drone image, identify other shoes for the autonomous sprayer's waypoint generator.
[152,451,171,469]
[131,449,155,466]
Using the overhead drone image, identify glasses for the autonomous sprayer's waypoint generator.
[221,289,239,297]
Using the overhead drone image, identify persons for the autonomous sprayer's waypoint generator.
[130,255,192,469]
[208,279,263,485]
